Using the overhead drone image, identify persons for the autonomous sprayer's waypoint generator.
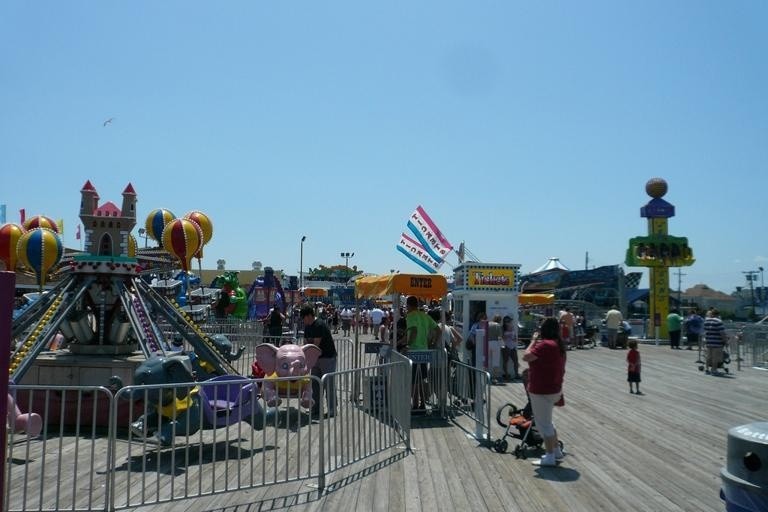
[406,298,440,414]
[666,307,684,350]
[521,316,568,467]
[351,308,373,334]
[704,305,714,319]
[625,340,644,396]
[341,305,353,337]
[270,306,285,347]
[265,304,277,319]
[683,310,704,350]
[318,304,338,334]
[369,305,393,342]
[554,305,576,351]
[428,309,463,404]
[702,308,729,377]
[604,304,622,350]
[501,316,521,379]
[487,314,503,383]
[471,312,488,369]
[299,307,338,418]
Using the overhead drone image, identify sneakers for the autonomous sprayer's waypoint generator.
[312,411,337,420]
[672,346,692,349]
[531,453,564,465]
[412,405,426,415]
[706,369,722,375]
[630,391,642,395]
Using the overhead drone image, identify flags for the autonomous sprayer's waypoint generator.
[406,203,454,264]
[394,232,445,274]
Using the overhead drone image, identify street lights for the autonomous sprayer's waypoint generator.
[340,252,355,285]
[759,267,764,287]
[300,235,306,292]
[744,271,760,317]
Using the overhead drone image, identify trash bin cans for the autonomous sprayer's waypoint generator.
[719,420,768,512]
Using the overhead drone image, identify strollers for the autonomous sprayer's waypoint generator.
[696,338,731,373]
[493,391,563,460]
